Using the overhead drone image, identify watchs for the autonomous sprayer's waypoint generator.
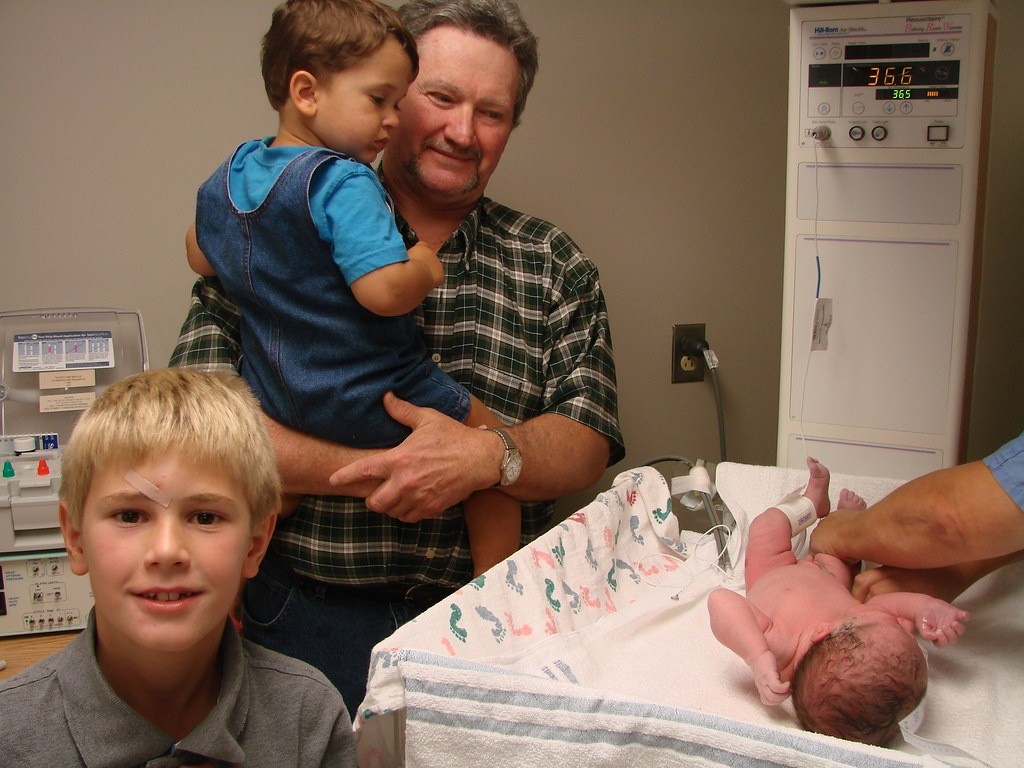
[486,426,522,490]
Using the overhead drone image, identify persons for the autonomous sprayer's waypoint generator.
[0,365,358,768]
[707,458,973,747]
[807,427,1024,604]
[166,0,626,729]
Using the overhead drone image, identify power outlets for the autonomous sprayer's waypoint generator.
[671,324,706,383]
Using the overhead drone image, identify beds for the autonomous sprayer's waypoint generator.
[355,461,1024,768]
